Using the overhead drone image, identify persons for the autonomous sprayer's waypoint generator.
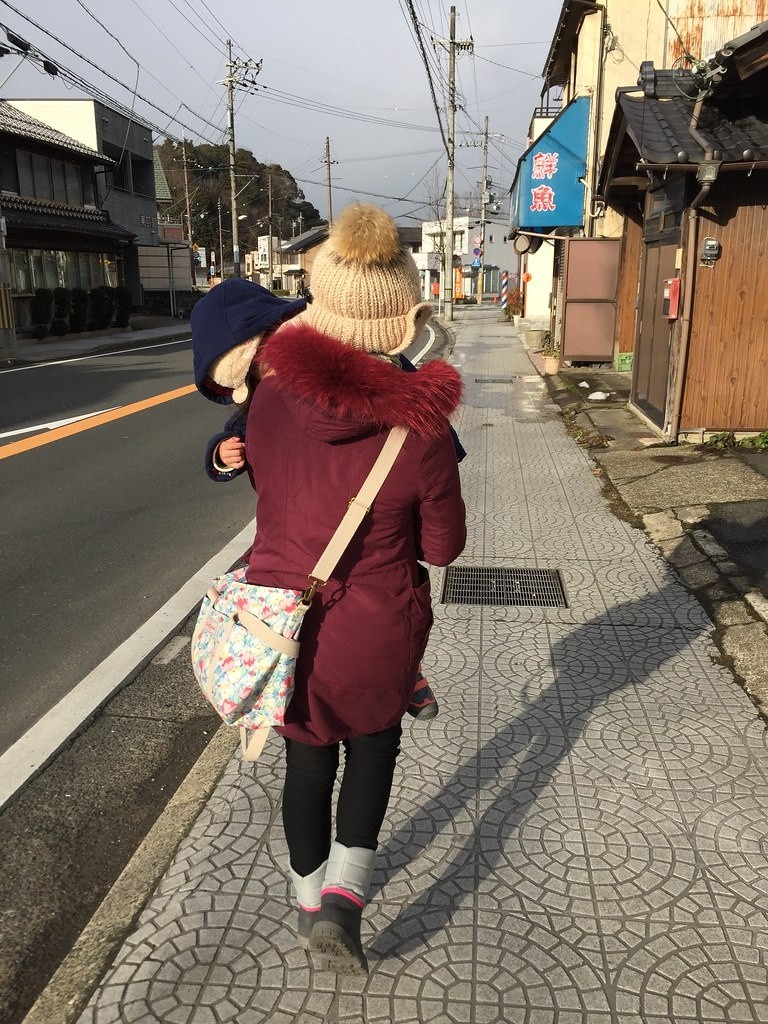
[189,280,466,722]
[245,203,467,978]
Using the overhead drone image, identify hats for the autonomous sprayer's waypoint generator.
[307,205,433,355]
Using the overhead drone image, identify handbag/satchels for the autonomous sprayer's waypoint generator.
[191,565,309,729]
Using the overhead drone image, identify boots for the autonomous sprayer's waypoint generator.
[288,853,327,949]
[310,841,376,979]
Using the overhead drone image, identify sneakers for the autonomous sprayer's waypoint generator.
[407,662,439,721]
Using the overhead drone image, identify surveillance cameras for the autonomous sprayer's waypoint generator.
[493,205,500,210]
